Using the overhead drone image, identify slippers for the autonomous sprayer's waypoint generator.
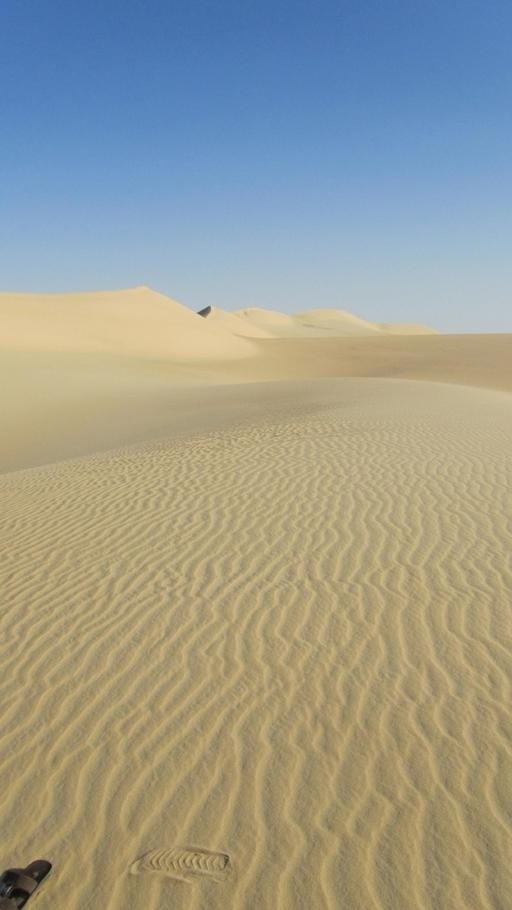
[0,860,52,910]
